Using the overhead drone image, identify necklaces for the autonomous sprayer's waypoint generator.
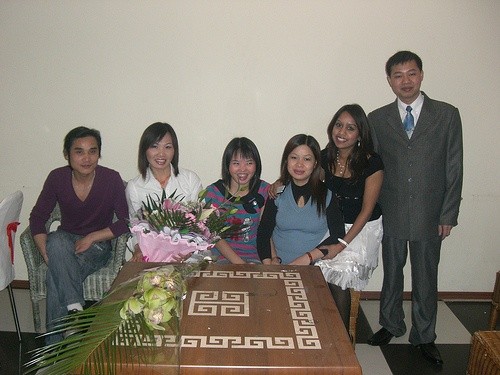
[335,151,344,175]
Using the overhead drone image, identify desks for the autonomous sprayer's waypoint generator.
[71,261,363,375]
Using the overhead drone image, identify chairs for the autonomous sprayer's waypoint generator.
[0,190,24,342]
[465,270,500,375]
[20,179,131,333]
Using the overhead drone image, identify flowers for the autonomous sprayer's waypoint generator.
[127,179,251,244]
[22,259,210,375]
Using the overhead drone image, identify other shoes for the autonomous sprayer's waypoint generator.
[409,342,443,366]
[368,326,394,346]
[63,310,85,339]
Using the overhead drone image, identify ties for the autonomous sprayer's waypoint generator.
[402,106,416,139]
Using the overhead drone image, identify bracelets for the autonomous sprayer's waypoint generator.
[306,252,313,265]
[160,174,170,186]
[272,256,282,264]
[338,238,349,247]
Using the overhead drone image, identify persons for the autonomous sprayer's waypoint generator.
[28,126,131,367]
[366,51,464,375]
[268,103,384,333]
[199,136,282,265]
[124,122,204,264]
[256,134,346,266]
